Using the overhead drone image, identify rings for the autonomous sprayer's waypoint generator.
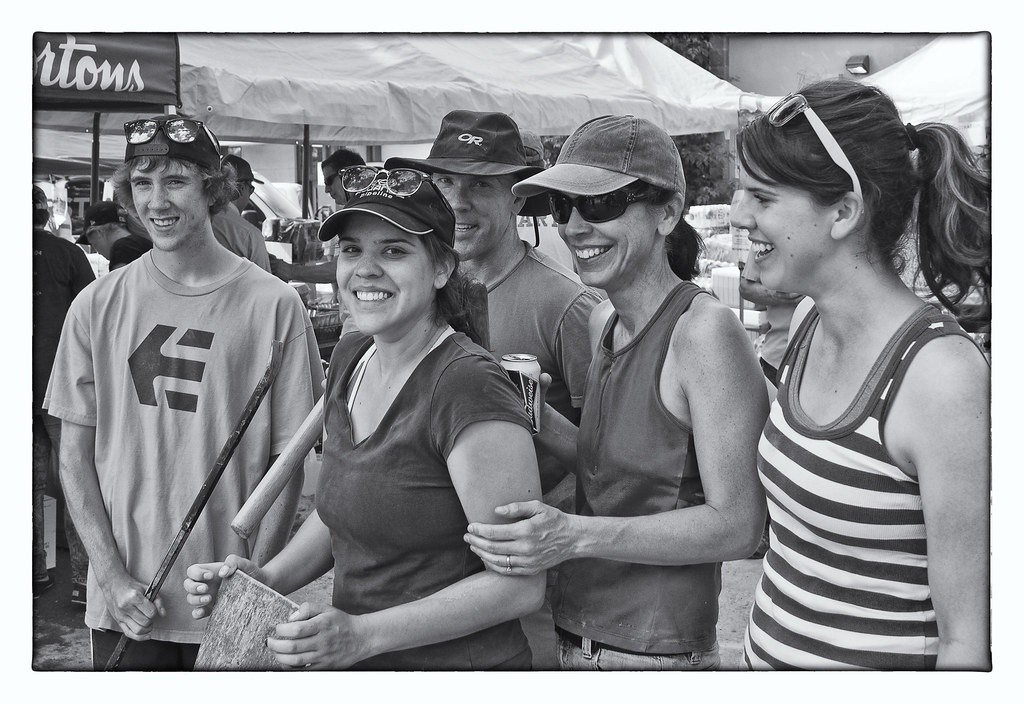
[506,555,512,572]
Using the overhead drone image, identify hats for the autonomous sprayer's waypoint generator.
[384,109,554,216]
[220,154,266,185]
[317,170,456,249]
[32,185,48,210]
[512,115,686,197]
[74,200,128,245]
[517,128,544,163]
[124,116,241,201]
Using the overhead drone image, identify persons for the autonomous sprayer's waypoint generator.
[385,108,607,423]
[738,243,806,388]
[463,114,765,672]
[36,115,365,672]
[184,150,548,672]
[726,77,989,671]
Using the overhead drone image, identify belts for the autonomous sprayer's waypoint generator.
[554,625,657,656]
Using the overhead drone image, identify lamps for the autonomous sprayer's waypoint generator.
[845,55,870,74]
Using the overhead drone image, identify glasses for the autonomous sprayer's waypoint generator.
[764,91,865,218]
[324,172,339,185]
[123,118,220,159]
[244,181,254,193]
[548,188,660,223]
[338,165,456,248]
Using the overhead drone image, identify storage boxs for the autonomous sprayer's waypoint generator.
[731,308,768,327]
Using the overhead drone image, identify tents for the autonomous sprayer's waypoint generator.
[33,35,776,218]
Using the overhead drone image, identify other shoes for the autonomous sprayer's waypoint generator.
[71,583,88,605]
[32,572,54,599]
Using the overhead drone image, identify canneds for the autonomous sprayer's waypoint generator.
[499,354,540,436]
